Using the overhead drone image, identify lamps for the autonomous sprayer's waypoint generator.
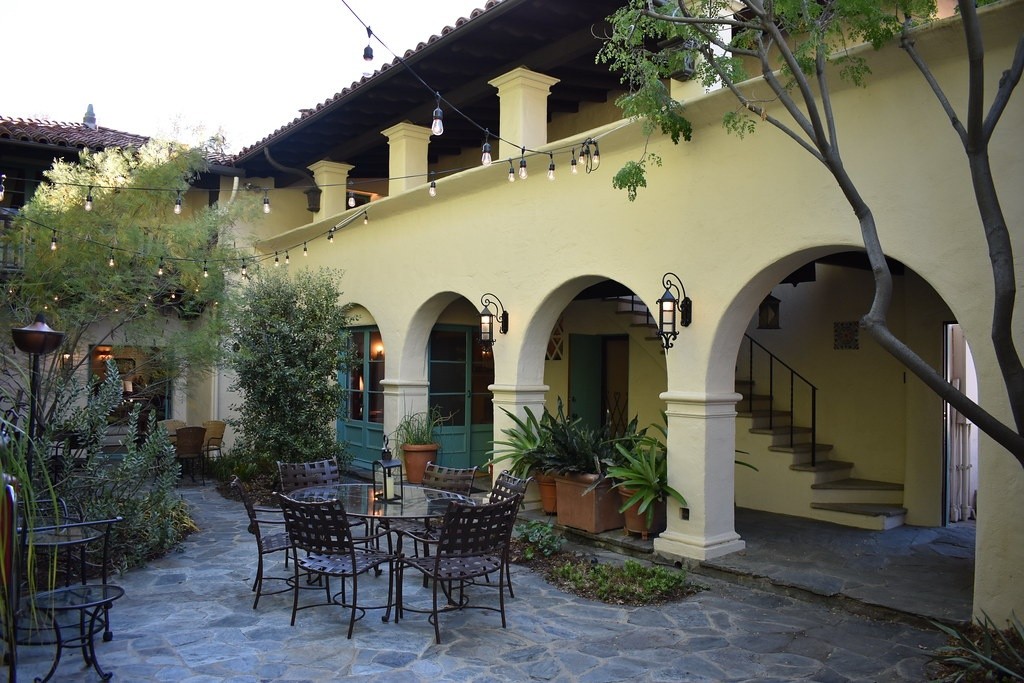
[477,293,509,356]
[654,272,692,350]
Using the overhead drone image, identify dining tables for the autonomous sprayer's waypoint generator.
[287,483,477,622]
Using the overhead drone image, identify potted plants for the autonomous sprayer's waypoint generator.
[481,395,687,540]
[386,405,457,482]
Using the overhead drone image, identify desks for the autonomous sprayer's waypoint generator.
[14,497,124,683]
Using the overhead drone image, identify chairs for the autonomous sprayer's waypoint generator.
[158,419,225,486]
[232,456,536,645]
